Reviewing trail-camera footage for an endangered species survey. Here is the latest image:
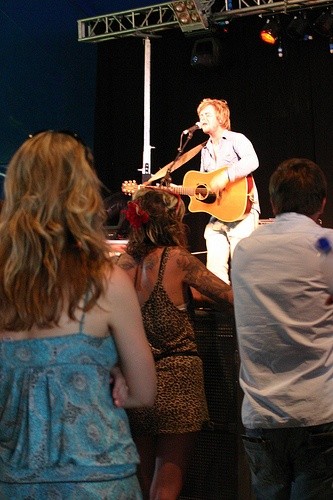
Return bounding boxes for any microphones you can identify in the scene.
[183,122,202,134]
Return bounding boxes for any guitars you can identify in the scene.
[121,163,255,222]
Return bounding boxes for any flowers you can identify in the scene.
[120,199,149,227]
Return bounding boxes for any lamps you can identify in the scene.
[169,0,210,33]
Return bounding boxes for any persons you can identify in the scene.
[156,98,261,286]
[231,160,333,500]
[0,132,156,500]
[116,188,234,500]
